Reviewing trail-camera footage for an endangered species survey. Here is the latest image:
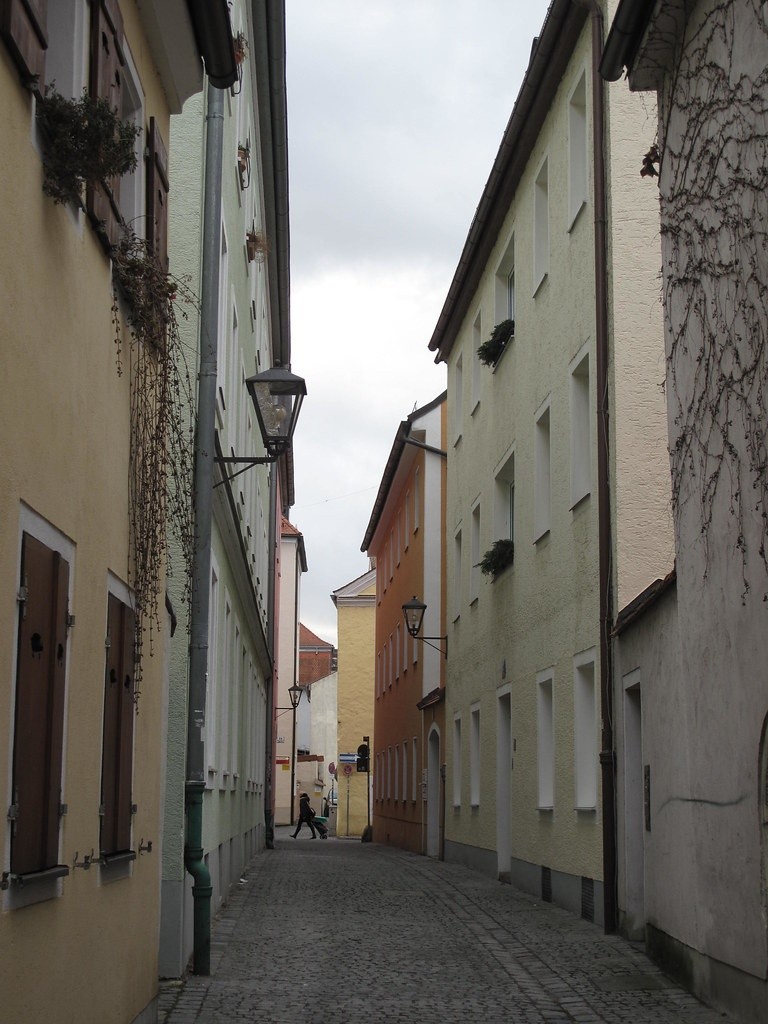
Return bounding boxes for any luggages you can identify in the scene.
[312,821,328,839]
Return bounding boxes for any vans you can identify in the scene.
[324,787,339,816]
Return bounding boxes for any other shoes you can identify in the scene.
[290,835,296,838]
[310,836,316,840]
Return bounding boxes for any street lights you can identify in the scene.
[287,681,302,825]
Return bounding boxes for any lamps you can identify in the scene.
[214,357,308,495]
[402,595,448,659]
[273,681,303,717]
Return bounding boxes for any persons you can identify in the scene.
[289,791,317,840]
